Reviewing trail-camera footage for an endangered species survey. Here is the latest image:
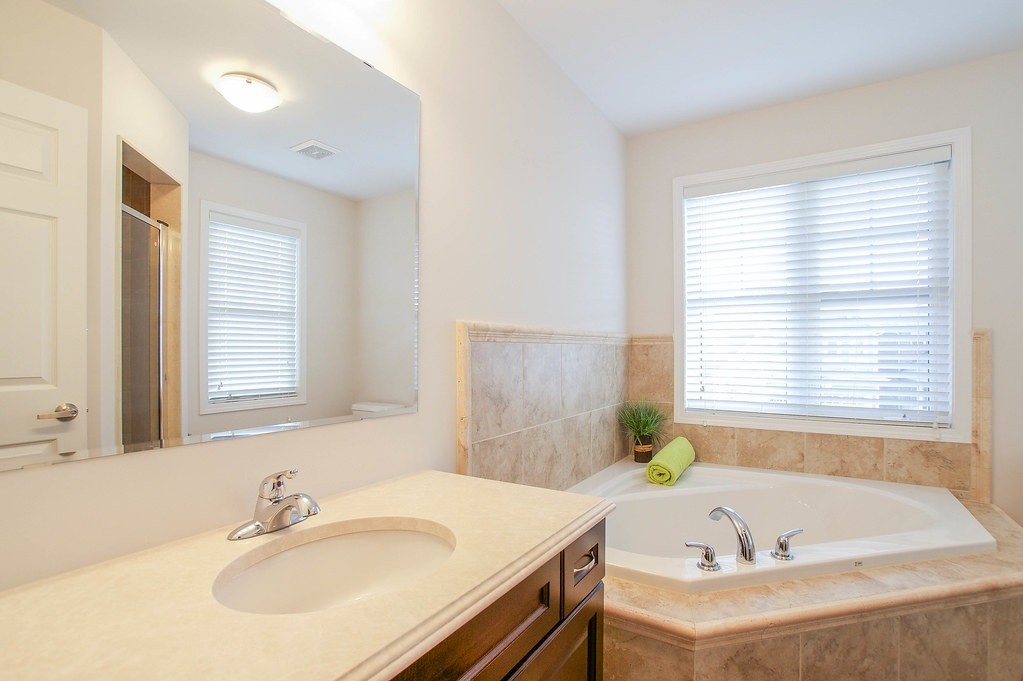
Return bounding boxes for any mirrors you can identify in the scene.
[0,0,424,470]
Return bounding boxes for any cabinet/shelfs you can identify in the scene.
[390,518,604,681]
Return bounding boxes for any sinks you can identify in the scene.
[211,516,456,614]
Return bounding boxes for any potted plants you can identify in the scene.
[617,396,669,464]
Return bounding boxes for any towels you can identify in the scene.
[646,436,695,485]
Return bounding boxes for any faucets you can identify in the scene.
[707,506,757,565]
[227,468,321,541]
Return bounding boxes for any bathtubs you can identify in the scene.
[561,455,997,594]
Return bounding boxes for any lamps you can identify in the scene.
[214,70,283,116]
